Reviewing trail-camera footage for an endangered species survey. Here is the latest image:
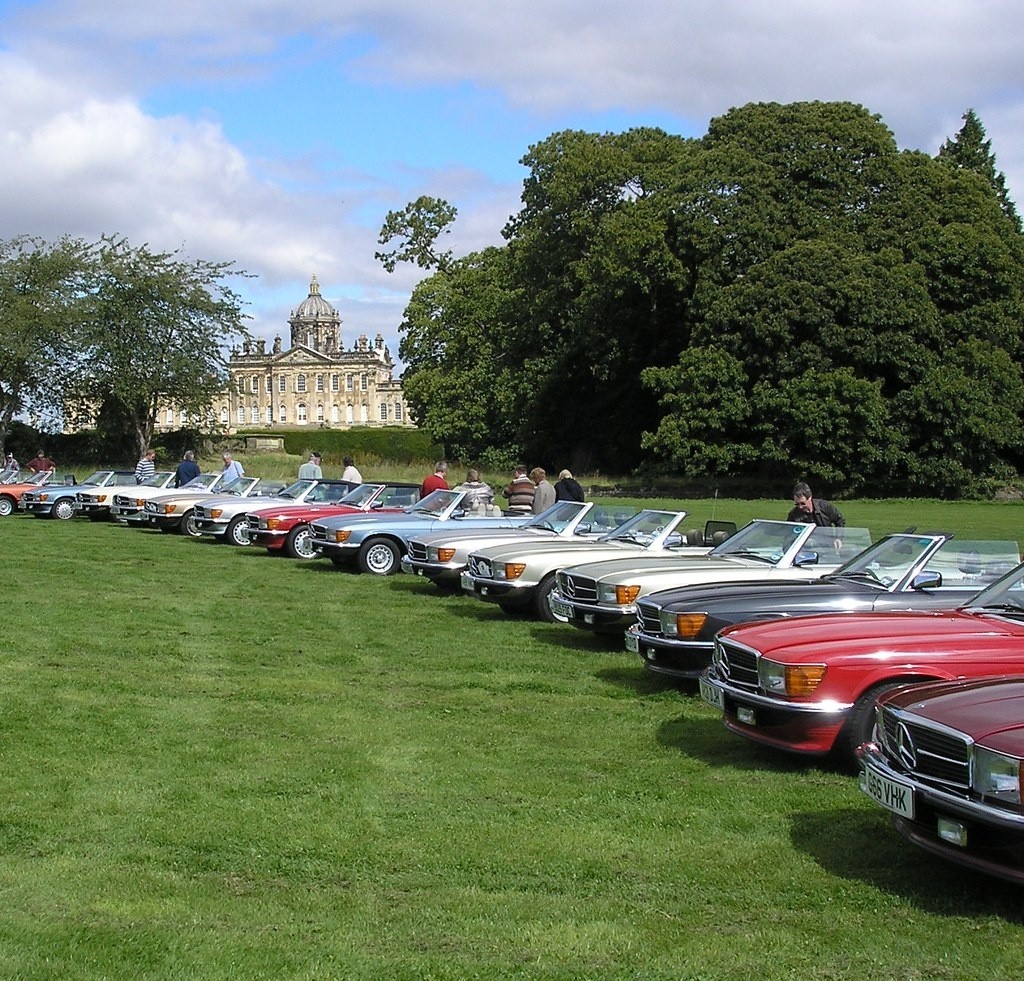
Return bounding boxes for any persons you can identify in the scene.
[26,450,55,472]
[2,452,20,471]
[420,461,449,508]
[342,457,362,484]
[783,482,846,556]
[134,449,155,483]
[458,469,495,508]
[175,451,200,487]
[502,464,584,521]
[222,452,245,483]
[298,452,326,497]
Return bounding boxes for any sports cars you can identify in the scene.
[459,507,783,623]
[547,520,912,641]
[624,526,1023,686]
[698,562,1024,774]
[0,459,605,620]
[855,675,1023,882]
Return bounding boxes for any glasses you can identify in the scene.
[794,502,806,505]
[152,456,155,458]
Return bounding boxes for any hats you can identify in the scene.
[310,451,320,458]
